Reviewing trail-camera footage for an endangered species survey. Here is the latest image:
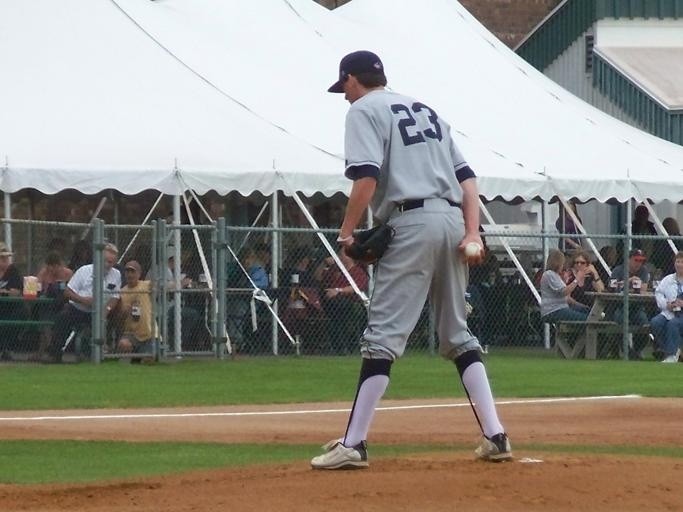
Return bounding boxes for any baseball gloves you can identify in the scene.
[345,224,394,262]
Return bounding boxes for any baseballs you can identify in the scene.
[465,242,480,257]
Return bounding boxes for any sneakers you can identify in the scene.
[475,433,512,461]
[28,352,62,361]
[119,358,131,362]
[311,438,370,470]
[631,347,645,359]
[661,348,681,363]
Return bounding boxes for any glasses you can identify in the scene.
[576,261,587,265]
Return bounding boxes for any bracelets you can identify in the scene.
[337,235,353,246]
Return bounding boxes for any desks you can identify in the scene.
[0,287,660,365]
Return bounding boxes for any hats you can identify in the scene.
[629,249,646,259]
[124,261,142,272]
[328,51,383,93]
[0,241,14,256]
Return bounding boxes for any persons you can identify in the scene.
[309,50,513,471]
[465,207,682,366]
[0,203,370,365]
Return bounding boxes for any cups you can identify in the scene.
[290,274,299,282]
[609,279,618,293]
[633,280,642,294]
